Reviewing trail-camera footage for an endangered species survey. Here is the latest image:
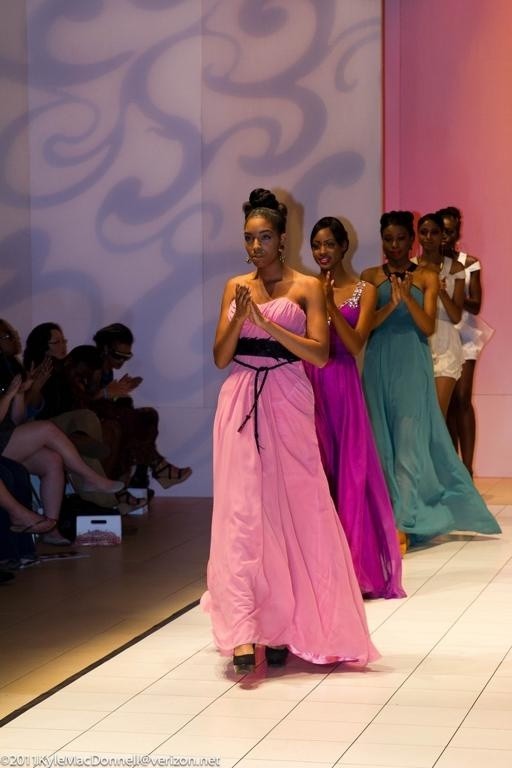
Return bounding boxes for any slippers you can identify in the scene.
[8,514,58,535]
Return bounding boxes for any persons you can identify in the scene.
[200,186,502,671]
[0,318,192,584]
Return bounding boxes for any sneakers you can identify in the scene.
[40,526,71,546]
[70,473,126,495]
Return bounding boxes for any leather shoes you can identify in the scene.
[232,643,256,675]
[264,644,289,665]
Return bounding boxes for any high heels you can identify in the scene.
[114,486,148,516]
[151,462,193,490]
